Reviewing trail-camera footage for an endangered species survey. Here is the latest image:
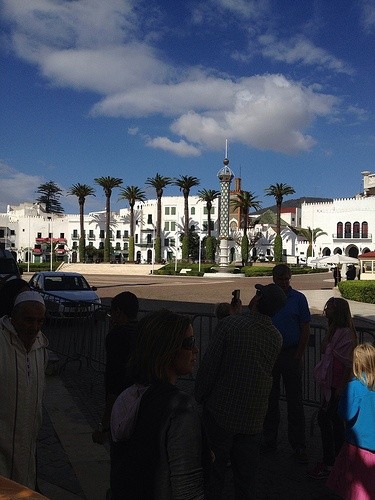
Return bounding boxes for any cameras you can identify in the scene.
[232,289,240,303]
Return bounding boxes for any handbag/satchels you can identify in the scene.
[109,385,150,443]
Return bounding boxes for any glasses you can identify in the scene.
[182,336,196,350]
[256,289,261,296]
[330,297,336,306]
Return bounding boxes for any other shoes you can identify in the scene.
[261,439,277,455]
[288,448,308,464]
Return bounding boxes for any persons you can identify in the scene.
[110,308,208,500]
[197,283,287,500]
[249,264,311,465]
[105,291,140,439]
[326,343,375,500]
[0,278,49,491]
[305,297,359,480]
[333,265,361,287]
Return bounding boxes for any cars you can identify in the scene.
[27,271,102,320]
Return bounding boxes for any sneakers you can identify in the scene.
[305,463,333,480]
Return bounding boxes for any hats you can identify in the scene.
[14,291,46,306]
[255,284,287,308]
